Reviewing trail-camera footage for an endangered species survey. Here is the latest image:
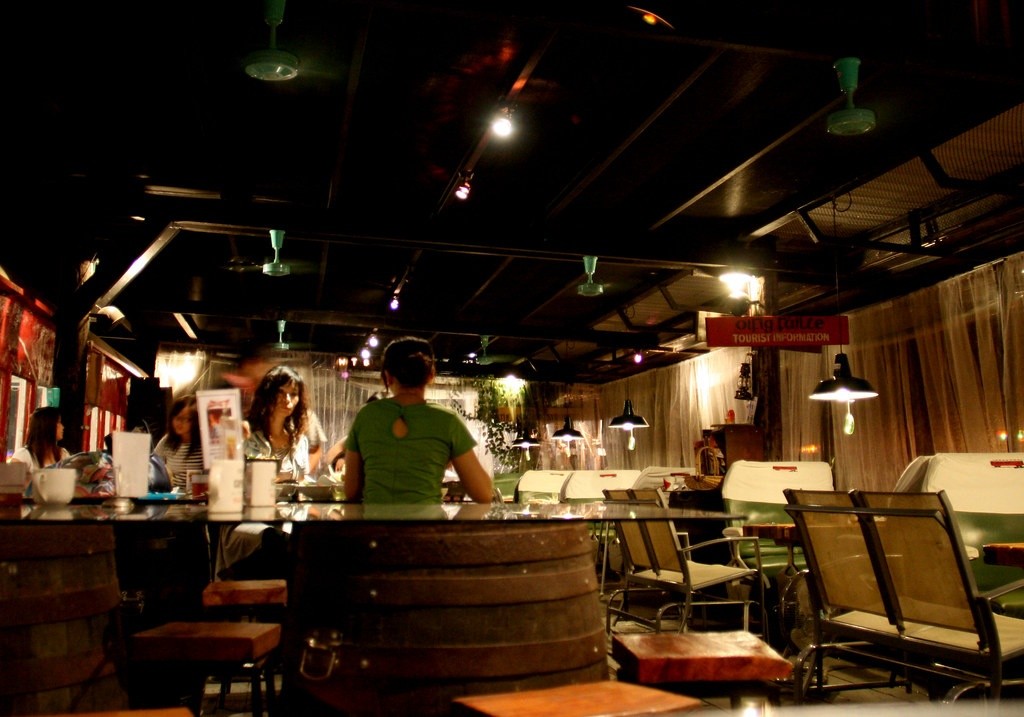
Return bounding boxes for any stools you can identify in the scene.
[611,631,793,711]
[131,578,290,717]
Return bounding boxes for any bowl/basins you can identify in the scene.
[293,482,332,500]
[276,483,298,502]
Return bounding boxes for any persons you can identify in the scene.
[216,366,327,625]
[344,338,492,504]
[342,504,491,520]
[154,396,210,619]
[333,451,347,471]
[11,406,70,496]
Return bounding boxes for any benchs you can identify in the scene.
[885,451,1024,620]
[721,460,844,616]
[513,466,695,544]
[783,488,1024,706]
[598,489,767,635]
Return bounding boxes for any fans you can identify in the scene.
[223,229,321,277]
[552,256,637,297]
[211,0,340,82]
[254,320,310,353]
[826,59,878,139]
[460,336,526,365]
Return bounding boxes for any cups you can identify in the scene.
[192,474,209,499]
[186,470,202,494]
[245,461,277,521]
[31,468,77,503]
[209,458,244,521]
[331,483,348,501]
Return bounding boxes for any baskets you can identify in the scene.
[686,447,723,490]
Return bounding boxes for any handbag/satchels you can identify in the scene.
[135,419,174,492]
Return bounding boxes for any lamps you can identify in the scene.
[809,190,879,435]
[93,303,126,332]
[734,352,755,402]
[551,406,585,457]
[512,417,541,461]
[391,297,399,309]
[608,371,649,451]
[456,179,471,200]
